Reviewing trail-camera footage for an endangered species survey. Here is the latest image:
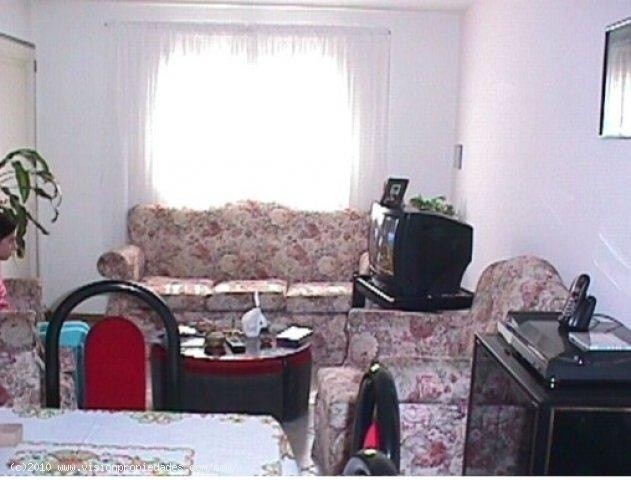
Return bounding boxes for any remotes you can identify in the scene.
[227,336,247,355]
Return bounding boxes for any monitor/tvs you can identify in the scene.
[367,201,474,301]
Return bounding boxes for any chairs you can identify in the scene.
[44,279,181,411]
[0,277,77,410]
[340,364,399,476]
[311,254,569,475]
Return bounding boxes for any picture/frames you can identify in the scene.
[380,179,409,208]
[598,16,631,139]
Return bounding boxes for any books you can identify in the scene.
[569,327,631,355]
[277,325,314,349]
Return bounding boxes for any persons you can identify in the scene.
[0,211,19,407]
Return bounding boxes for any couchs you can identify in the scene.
[98,205,369,366]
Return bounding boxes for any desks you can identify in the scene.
[0,407,297,476]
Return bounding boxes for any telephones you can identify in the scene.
[557,274,597,332]
